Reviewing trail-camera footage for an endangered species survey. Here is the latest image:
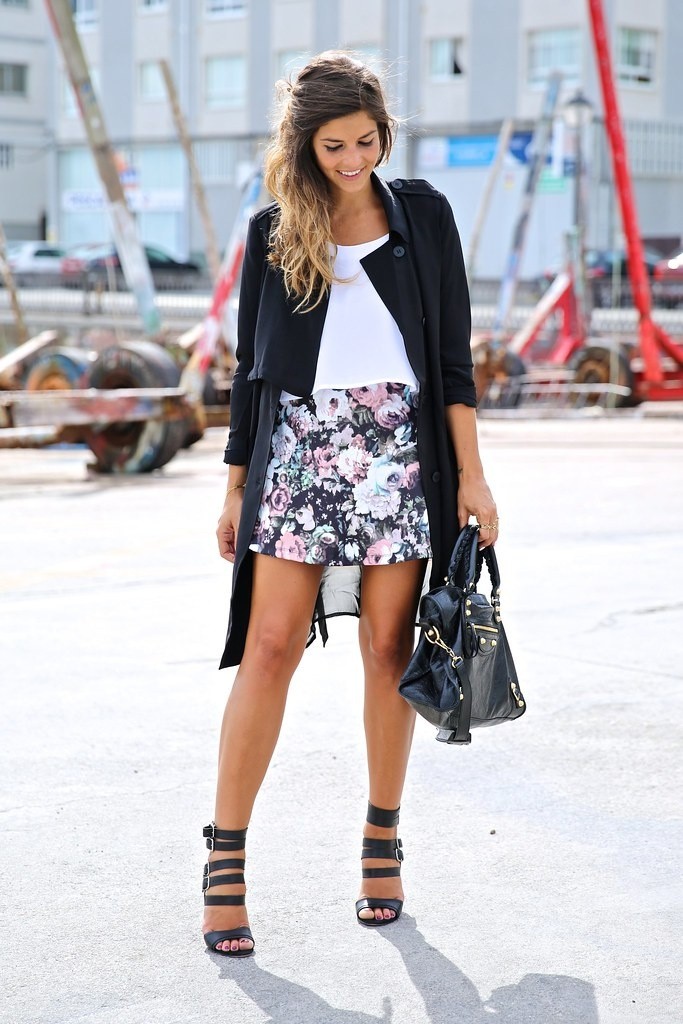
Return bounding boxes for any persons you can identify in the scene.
[200,52,500,959]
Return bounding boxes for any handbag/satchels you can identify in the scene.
[396,524,526,745]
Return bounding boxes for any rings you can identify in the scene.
[479,522,497,531]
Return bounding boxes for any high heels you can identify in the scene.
[203,821,255,958]
[356,801,404,927]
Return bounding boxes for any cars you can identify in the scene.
[541,250,662,308]
[0,240,71,287]
[62,242,199,293]
[653,248,683,283]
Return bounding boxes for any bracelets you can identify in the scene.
[225,483,246,497]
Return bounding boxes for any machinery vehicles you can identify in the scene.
[0,0,683,473]
